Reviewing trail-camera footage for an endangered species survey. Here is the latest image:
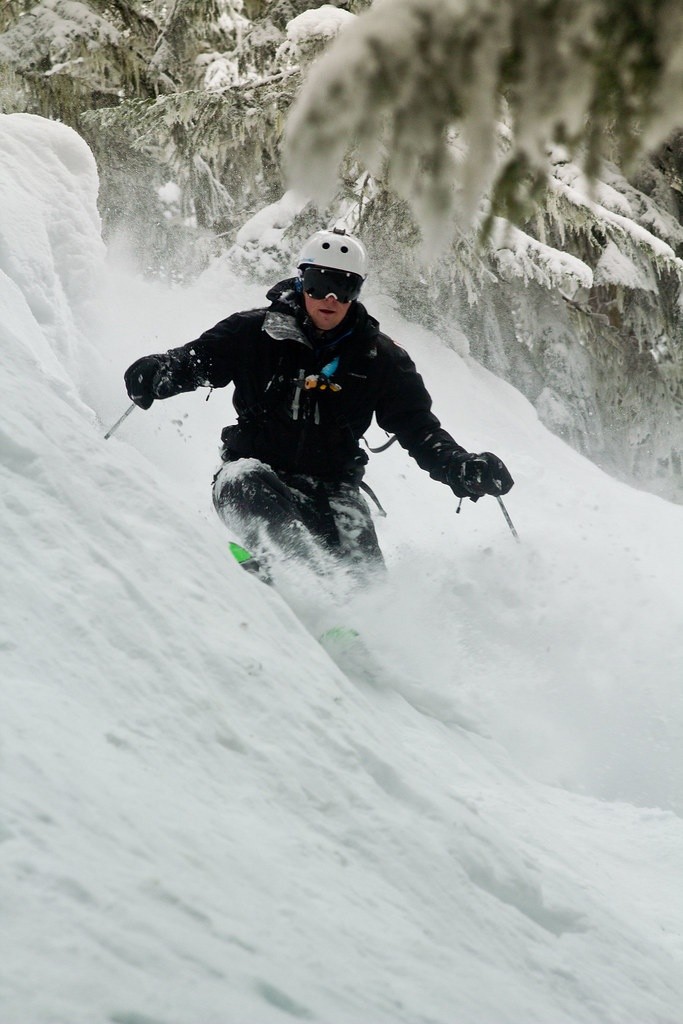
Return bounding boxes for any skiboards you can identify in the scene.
[229,540,279,592]
[315,622,386,682]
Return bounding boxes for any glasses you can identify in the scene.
[299,268,364,304]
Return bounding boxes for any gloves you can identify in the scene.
[123,357,171,410]
[448,447,515,515]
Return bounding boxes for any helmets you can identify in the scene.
[296,226,368,280]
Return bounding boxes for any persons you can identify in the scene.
[125,228,514,591]
